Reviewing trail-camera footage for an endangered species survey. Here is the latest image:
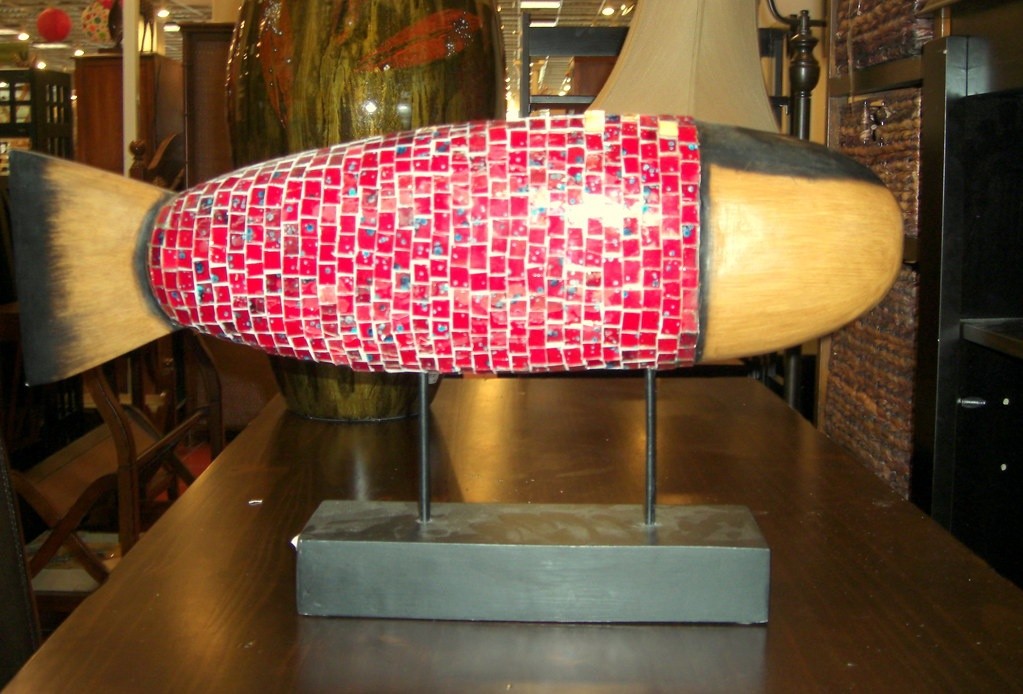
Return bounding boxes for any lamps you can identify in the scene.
[602,1,614,16]
[584,0,780,135]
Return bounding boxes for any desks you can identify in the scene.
[0,376,1023,694]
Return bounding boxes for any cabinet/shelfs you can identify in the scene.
[0,52,158,182]
